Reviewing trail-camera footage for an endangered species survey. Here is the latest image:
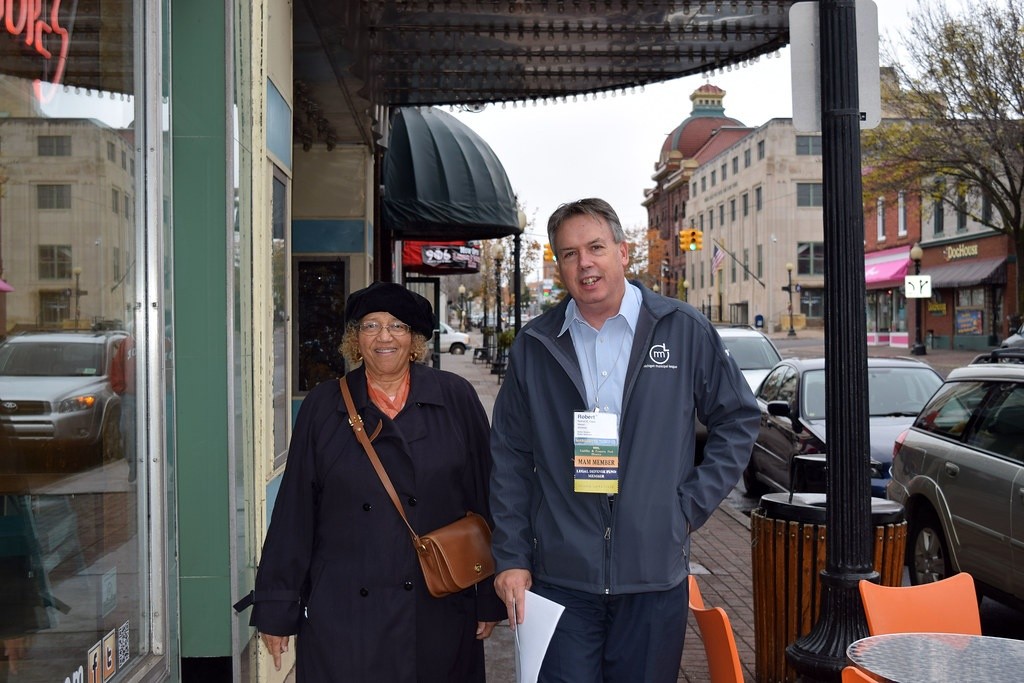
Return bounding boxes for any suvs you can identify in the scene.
[711,322,784,395]
[0,329,131,462]
[886,347,1024,611]
[741,357,974,498]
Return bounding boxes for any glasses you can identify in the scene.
[354,319,412,337]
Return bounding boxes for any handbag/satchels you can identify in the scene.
[417,512,496,598]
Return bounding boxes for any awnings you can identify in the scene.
[401,240,483,275]
[918,256,1008,288]
[386,104,519,240]
[864,244,913,288]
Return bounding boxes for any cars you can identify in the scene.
[427,322,472,356]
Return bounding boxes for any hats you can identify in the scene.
[345,280,434,342]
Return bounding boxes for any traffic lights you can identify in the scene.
[688,230,697,251]
[679,230,688,251]
[696,231,704,250]
[544,242,554,262]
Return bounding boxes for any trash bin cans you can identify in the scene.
[755,314,764,331]
[781,312,807,331]
[749,492,908,683]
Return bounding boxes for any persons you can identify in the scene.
[0,402,54,682]
[111,319,170,483]
[250,281,508,683]
[487,197,761,683]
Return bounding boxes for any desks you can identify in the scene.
[846,633,1024,683]
[30,456,136,640]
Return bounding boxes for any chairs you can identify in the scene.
[987,405,1024,454]
[858,572,983,635]
[689,574,744,683]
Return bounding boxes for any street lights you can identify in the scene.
[910,242,926,354]
[785,263,796,336]
[458,284,466,333]
[74,267,82,329]
[490,243,508,374]
[513,202,527,337]
